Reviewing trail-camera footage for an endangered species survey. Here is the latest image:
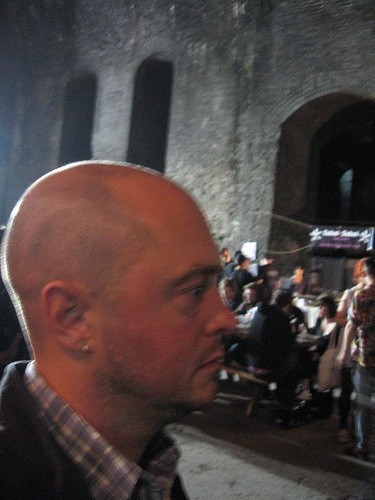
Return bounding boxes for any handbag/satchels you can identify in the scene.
[317,318,346,392]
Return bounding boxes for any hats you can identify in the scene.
[269,289,293,310]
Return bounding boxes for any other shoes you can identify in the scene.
[338,427,350,443]
[311,411,331,421]
[295,384,313,401]
[271,398,306,418]
[344,445,367,459]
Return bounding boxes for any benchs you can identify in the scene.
[218,363,268,416]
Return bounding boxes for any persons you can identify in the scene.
[0,159,237,500]
[217,240,375,463]
